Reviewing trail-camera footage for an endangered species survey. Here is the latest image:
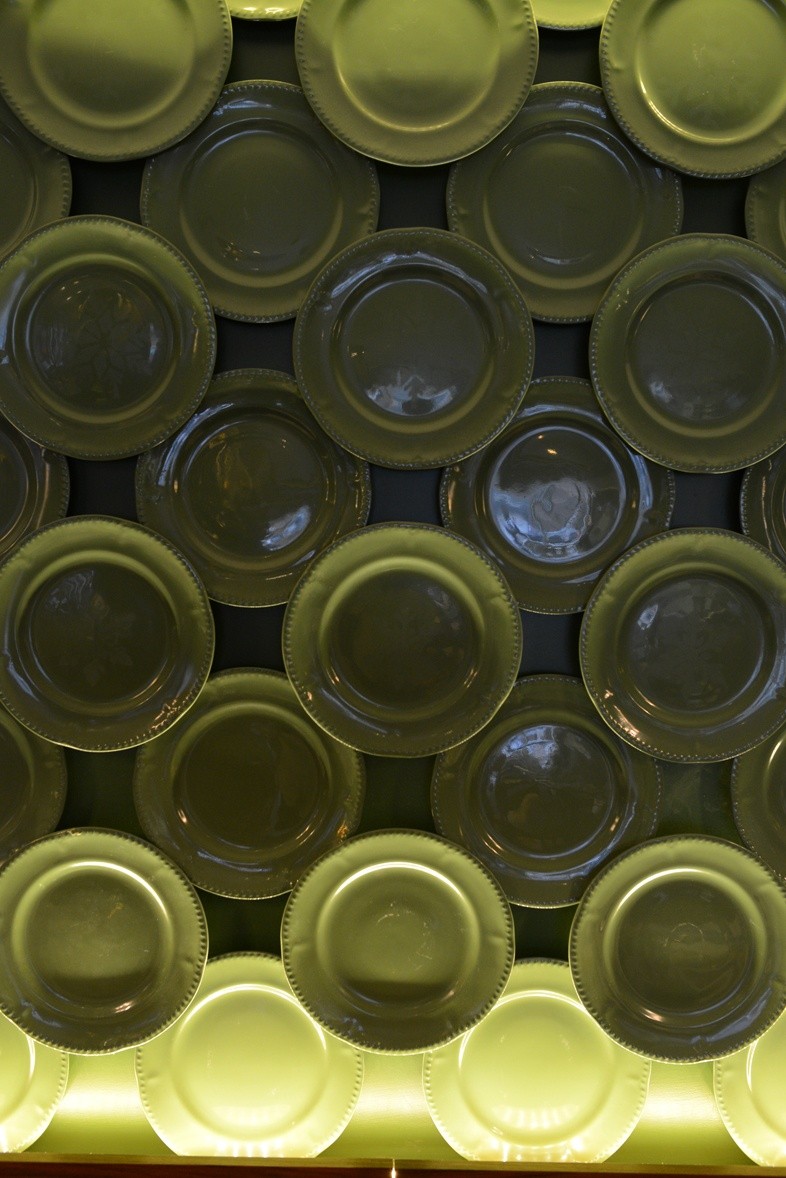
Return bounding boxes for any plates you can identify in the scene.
[139,78,381,322]
[291,227,537,469]
[422,958,651,1163]
[0,0,785,177]
[445,80,683,325]
[429,675,663,909]
[279,827,516,1057]
[440,376,676,615]
[135,951,364,1159]
[132,667,365,901]
[280,521,523,758]
[0,93,216,1155]
[713,723,786,1169]
[568,833,786,1065]
[589,161,786,565]
[578,526,786,764]
[133,368,371,608]
